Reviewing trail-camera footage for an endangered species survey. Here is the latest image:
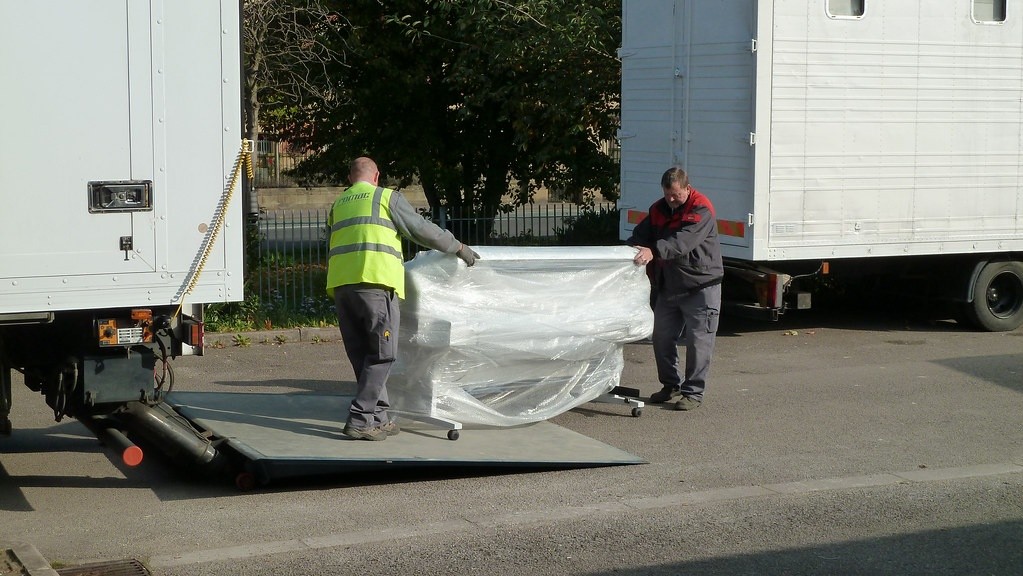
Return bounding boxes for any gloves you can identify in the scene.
[456,243,481,268]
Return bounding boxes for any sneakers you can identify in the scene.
[343,421,387,441]
[373,420,399,436]
[649,385,683,403]
[674,396,700,411]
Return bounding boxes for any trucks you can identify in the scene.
[615,0,1023,332]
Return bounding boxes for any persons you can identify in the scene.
[325,156,479,442]
[623,167,723,410]
